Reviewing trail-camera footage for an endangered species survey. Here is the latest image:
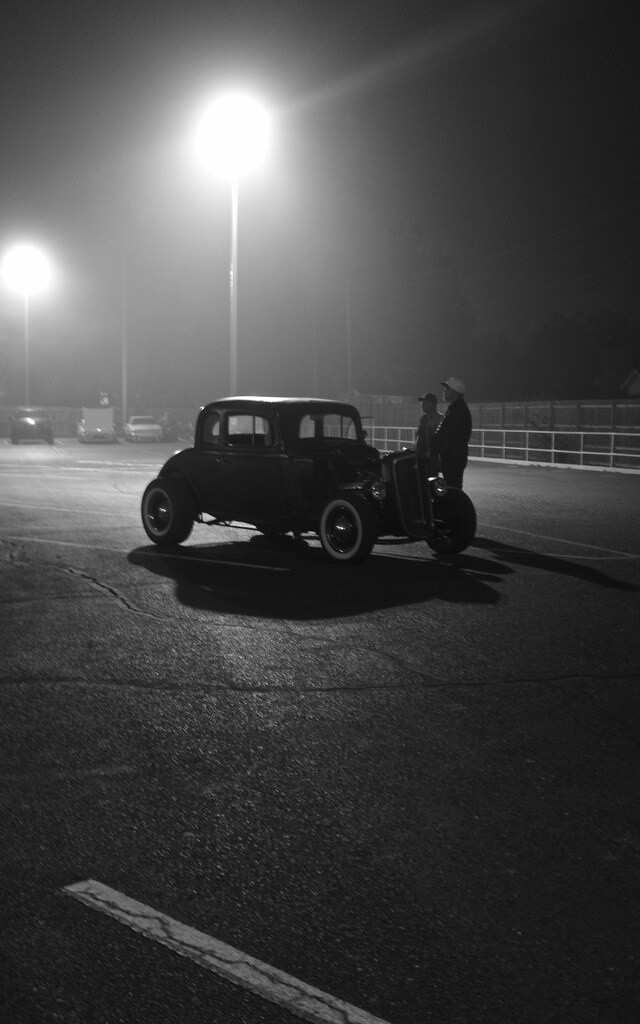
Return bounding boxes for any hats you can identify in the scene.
[440,377,465,394]
[418,393,437,401]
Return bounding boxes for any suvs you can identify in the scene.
[9,408,55,445]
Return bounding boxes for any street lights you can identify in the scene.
[1,243,51,406]
[184,85,272,395]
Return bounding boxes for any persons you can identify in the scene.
[414,392,445,477]
[429,376,472,490]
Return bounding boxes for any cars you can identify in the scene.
[124,415,162,443]
[77,406,117,444]
[141,394,480,564]
[159,413,193,439]
